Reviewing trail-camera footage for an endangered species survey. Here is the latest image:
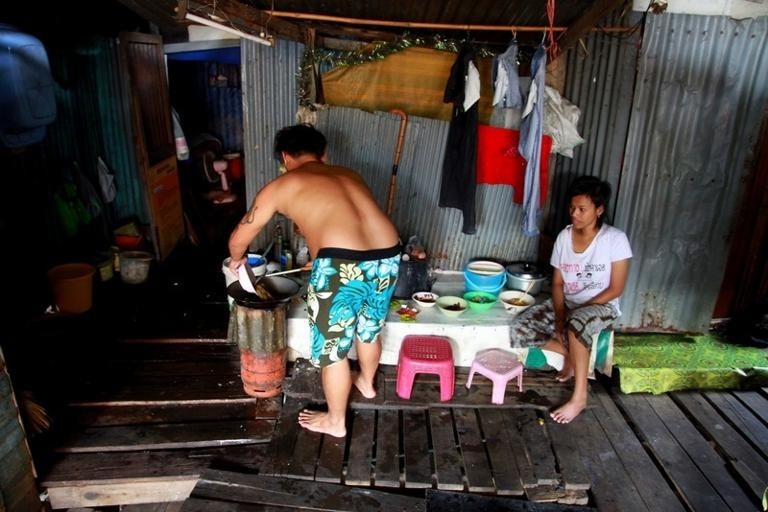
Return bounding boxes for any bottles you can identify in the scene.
[285,251,292,270]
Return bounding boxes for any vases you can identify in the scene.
[47,250,154,315]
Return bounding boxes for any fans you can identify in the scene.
[198,145,238,204]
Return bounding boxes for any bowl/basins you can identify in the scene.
[239,263,258,294]
[412,290,536,318]
[114,233,143,248]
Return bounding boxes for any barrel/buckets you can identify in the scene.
[463,261,507,299]
[48,262,95,314]
[221,254,267,312]
[392,261,428,301]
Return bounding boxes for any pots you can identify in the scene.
[227,275,299,309]
[506,264,549,296]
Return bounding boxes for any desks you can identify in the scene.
[285,299,552,366]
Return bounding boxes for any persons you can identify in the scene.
[228,122,402,437]
[509,175,634,424]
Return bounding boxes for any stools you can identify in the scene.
[395,335,525,404]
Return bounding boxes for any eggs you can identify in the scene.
[418,251,426,259]
